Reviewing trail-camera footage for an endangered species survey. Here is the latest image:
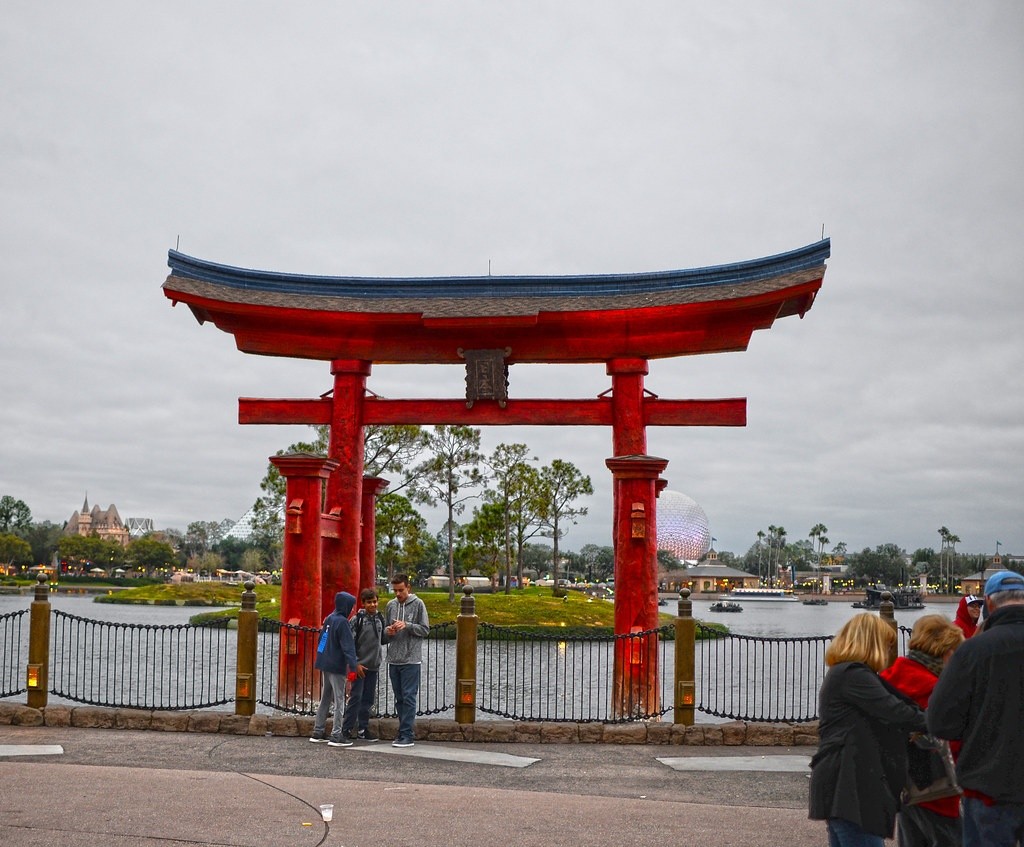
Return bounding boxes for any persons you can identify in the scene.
[309,591,367,746]
[385,573,430,747]
[235,569,245,581]
[878,613,967,847]
[341,588,388,743]
[808,612,928,847]
[950,593,985,640]
[928,570,1024,847]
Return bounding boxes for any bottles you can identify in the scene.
[265,729,272,747]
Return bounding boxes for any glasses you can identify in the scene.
[970,604,982,608]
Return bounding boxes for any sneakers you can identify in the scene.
[327,736,354,746]
[357,729,379,742]
[309,735,330,742]
[391,736,414,747]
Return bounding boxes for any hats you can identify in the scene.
[983,572,1024,597]
[965,595,984,605]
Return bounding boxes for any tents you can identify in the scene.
[465,577,490,587]
[427,576,450,589]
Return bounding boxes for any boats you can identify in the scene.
[709,602,744,612]
[803,598,827,606]
[850,584,927,610]
[658,597,668,606]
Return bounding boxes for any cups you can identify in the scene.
[320,804,334,821]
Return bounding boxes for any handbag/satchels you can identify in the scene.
[901,732,962,805]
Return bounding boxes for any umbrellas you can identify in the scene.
[30,564,54,573]
[90,567,105,575]
[115,568,125,573]
